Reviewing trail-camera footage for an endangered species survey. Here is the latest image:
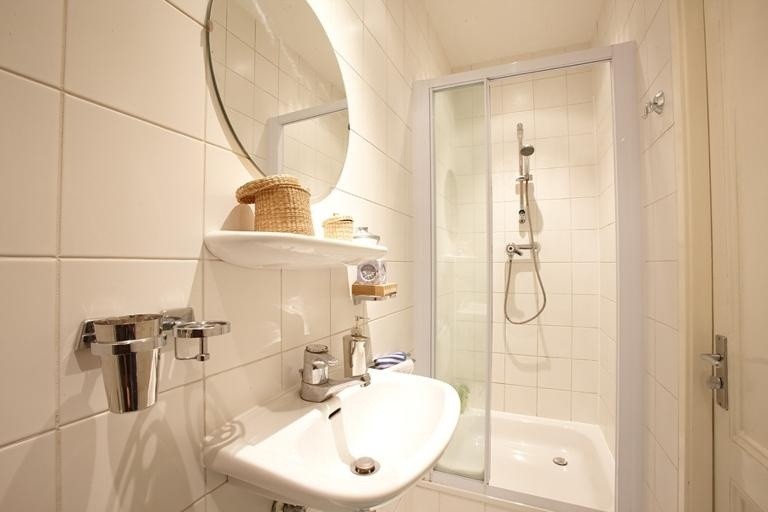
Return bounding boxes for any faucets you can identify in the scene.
[300,344,375,401]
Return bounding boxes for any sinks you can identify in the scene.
[203,368,462,510]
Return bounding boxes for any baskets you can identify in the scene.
[322,215,355,240]
[235,175,315,234]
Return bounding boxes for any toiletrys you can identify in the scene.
[341,316,369,378]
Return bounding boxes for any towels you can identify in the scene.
[372,351,414,376]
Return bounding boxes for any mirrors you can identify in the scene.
[206,0,350,206]
